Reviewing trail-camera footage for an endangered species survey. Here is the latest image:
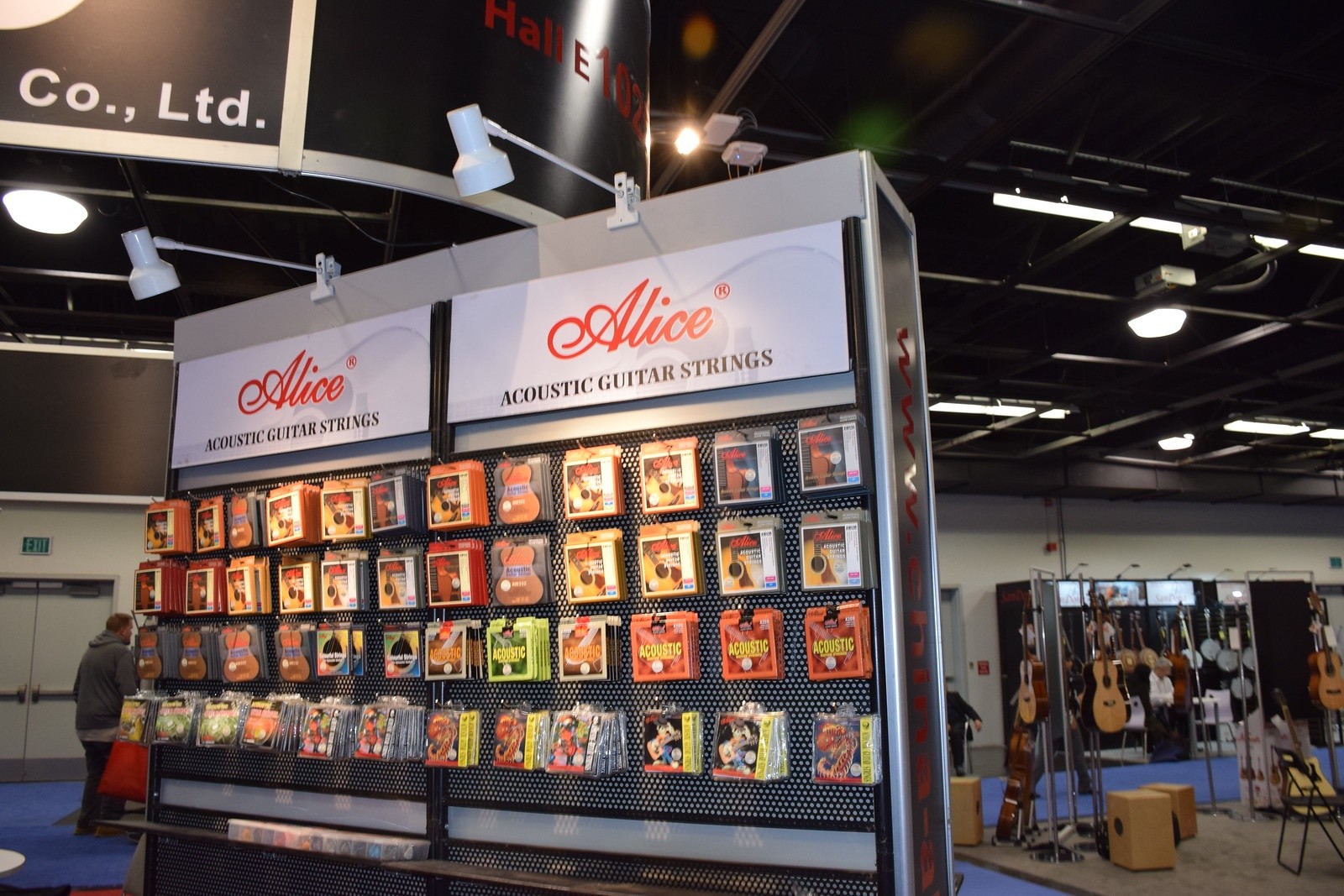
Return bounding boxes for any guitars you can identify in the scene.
[1270,685,1339,816]
[1017,587,1052,725]
[1100,594,1133,727]
[1118,600,1261,712]
[1081,588,1127,736]
[994,719,1039,844]
[1306,588,1344,711]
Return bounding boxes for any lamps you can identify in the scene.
[447,102,642,229]
[121,225,341,301]
[2,189,88,233]
[928,192,1344,451]
[673,118,708,155]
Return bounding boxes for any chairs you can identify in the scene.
[1271,746,1344,877]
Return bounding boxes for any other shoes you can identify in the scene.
[74,827,92,835]
[956,766,965,776]
[94,824,126,837]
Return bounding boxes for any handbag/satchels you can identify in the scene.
[96,739,149,804]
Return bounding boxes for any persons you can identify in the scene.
[944,677,982,777]
[1149,657,1175,731]
[1018,652,1099,800]
[1104,584,1128,607]
[72,613,140,836]
